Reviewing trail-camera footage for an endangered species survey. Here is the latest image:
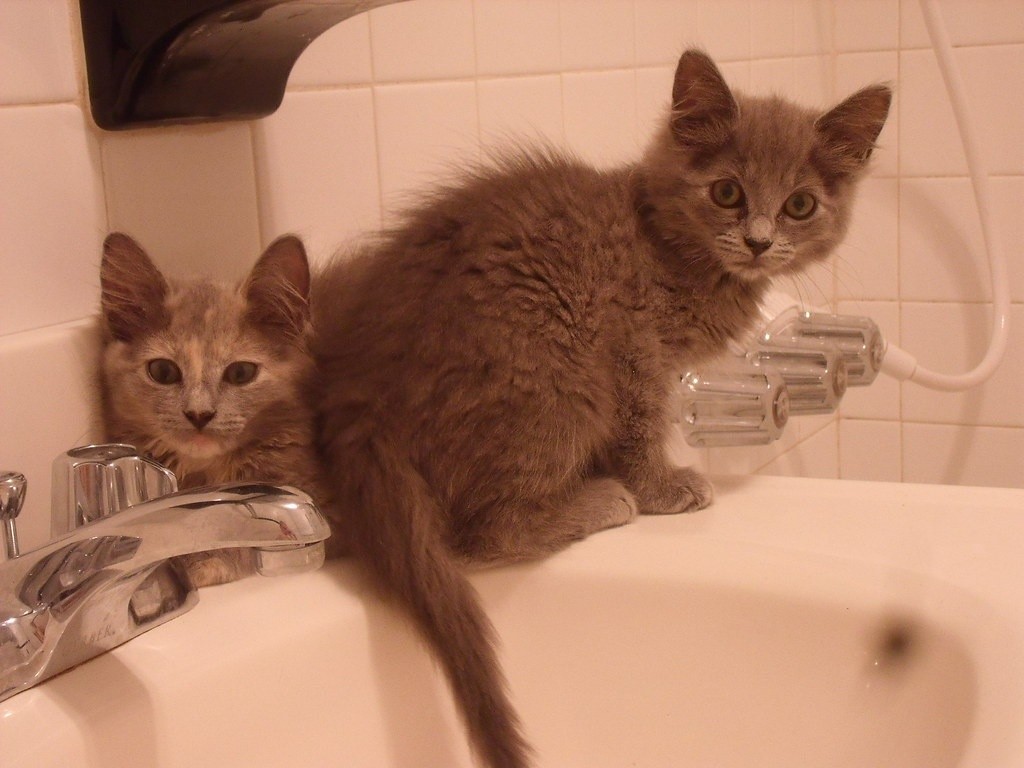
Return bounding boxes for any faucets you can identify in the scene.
[1,476,330,694]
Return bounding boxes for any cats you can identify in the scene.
[310,46,897,768]
[88,228,310,583]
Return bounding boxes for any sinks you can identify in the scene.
[0,469,1023,768]
